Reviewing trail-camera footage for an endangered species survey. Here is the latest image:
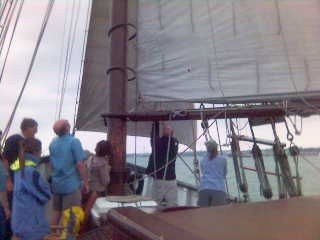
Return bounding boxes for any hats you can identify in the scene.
[205,141,218,149]
[53,120,70,136]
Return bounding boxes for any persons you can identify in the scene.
[142,109,179,211]
[0,131,22,222]
[199,139,227,207]
[11,138,56,240]
[84,140,111,199]
[49,119,88,232]
[21,118,40,151]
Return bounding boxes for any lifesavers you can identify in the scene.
[126,171,145,195]
